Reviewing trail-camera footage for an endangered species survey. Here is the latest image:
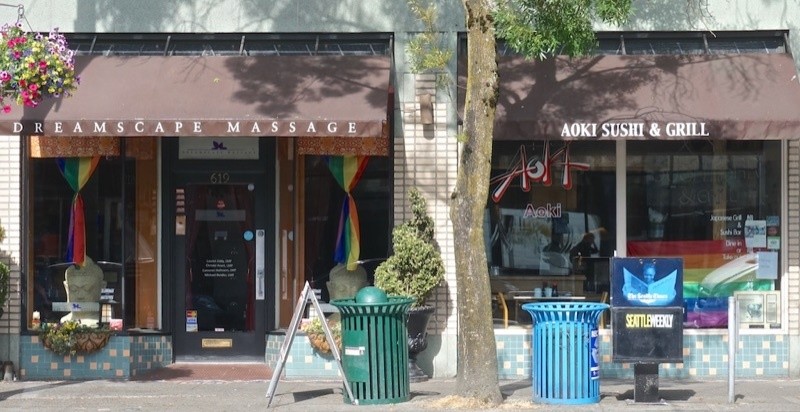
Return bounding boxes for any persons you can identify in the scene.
[569,233,599,289]
[622,263,677,304]
[59,255,107,327]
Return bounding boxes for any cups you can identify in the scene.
[552,286,558,297]
[534,286,543,297]
[544,286,553,297]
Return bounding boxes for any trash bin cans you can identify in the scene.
[329,287,416,405]
[522,301,610,406]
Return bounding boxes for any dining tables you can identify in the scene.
[510,287,585,324]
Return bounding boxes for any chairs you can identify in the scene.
[596,290,609,327]
[492,291,515,328]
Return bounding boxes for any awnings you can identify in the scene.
[455,52,799,140]
[0,56,391,137]
